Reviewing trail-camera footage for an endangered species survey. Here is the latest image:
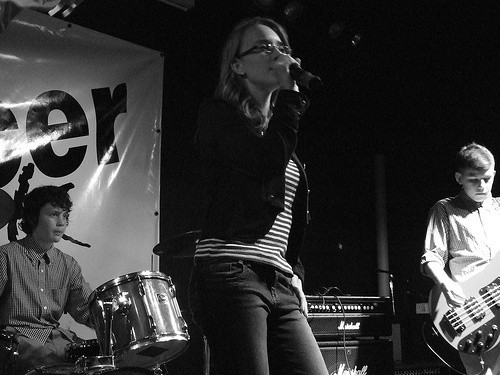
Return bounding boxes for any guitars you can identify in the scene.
[428,273,500,355]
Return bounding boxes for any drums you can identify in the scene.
[87,365,164,375]
[86,271,191,368]
[0,329,19,375]
[24,364,89,375]
[63,339,104,373]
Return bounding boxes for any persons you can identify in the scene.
[187,16,329,375]
[0,185,94,375]
[420,142,500,375]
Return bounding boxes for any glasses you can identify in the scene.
[238,43,292,60]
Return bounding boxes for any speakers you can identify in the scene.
[316,339,396,375]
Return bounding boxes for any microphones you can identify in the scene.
[289,64,323,92]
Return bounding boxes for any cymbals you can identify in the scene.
[153,229,201,256]
